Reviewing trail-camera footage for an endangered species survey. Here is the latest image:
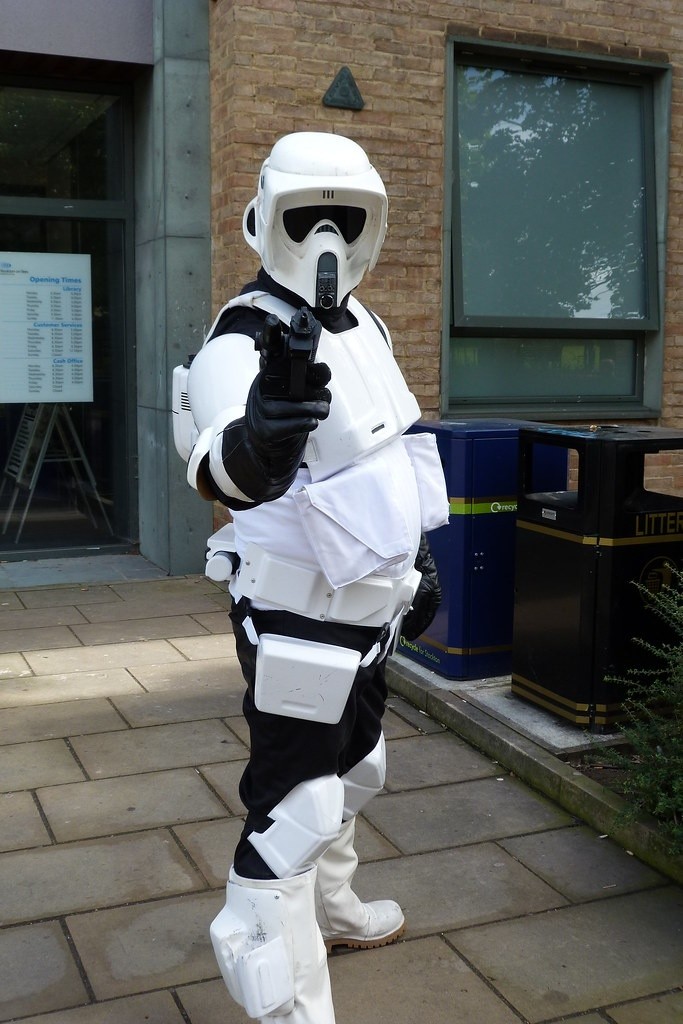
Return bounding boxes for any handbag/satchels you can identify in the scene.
[240,608,406,725]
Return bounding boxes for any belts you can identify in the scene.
[233,542,422,628]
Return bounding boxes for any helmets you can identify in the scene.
[242,131,390,312]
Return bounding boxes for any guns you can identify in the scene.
[254,306,323,401]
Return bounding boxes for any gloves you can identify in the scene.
[220,340,332,504]
[397,531,442,641]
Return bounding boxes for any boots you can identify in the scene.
[210,860,336,1024]
[313,813,406,953]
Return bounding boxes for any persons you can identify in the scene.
[187,132,450,1024]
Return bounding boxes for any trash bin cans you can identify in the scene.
[512,423,683,731]
[401,419,569,683]
